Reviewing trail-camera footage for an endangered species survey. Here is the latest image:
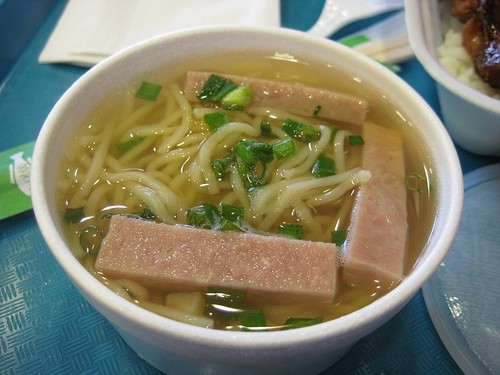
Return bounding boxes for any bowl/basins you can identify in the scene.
[29,24,464,375]
[405,0,500,157]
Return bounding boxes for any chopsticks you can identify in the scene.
[348,35,413,65]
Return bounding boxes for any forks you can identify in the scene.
[304,0,403,40]
